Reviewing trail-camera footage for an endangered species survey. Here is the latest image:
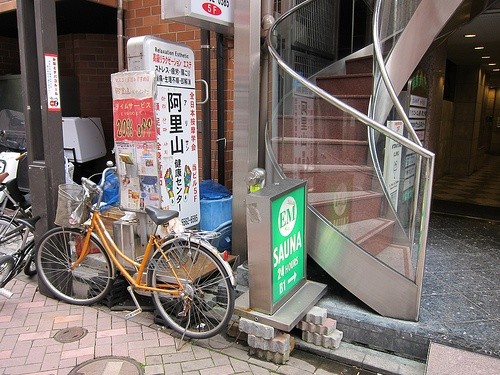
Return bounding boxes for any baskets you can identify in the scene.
[53,184,91,227]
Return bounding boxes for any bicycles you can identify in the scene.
[0,213,40,288]
[34,161,238,351]
[0,170,32,248]
